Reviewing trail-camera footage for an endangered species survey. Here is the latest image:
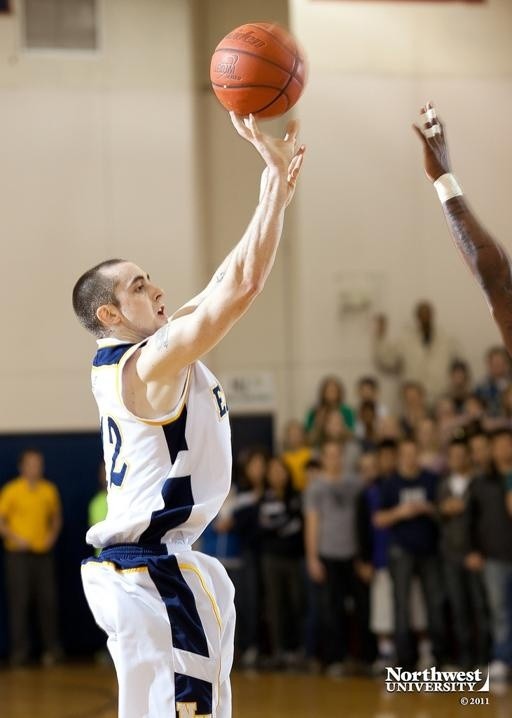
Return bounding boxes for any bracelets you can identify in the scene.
[431,171,464,202]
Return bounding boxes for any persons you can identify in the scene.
[0,453,60,671]
[72,112,307,718]
[410,100,512,351]
[82,457,114,665]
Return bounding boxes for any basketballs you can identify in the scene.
[209,22,308,121]
